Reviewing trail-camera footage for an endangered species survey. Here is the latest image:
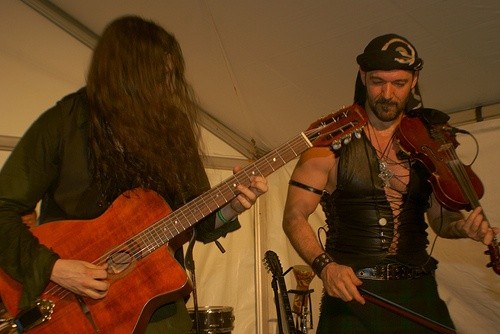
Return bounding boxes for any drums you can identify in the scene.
[187,305,235,334]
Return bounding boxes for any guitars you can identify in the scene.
[261,248,298,334]
[0,102,371,334]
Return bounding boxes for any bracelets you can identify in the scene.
[217,210,229,224]
[311,252,337,279]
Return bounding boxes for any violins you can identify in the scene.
[396,106,500,276]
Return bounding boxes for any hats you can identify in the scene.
[356,33,424,73]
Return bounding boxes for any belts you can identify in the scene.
[357,263,433,282]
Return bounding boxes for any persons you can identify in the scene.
[282,34,500,334]
[0,14,269,334]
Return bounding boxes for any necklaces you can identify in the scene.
[368,120,397,187]
